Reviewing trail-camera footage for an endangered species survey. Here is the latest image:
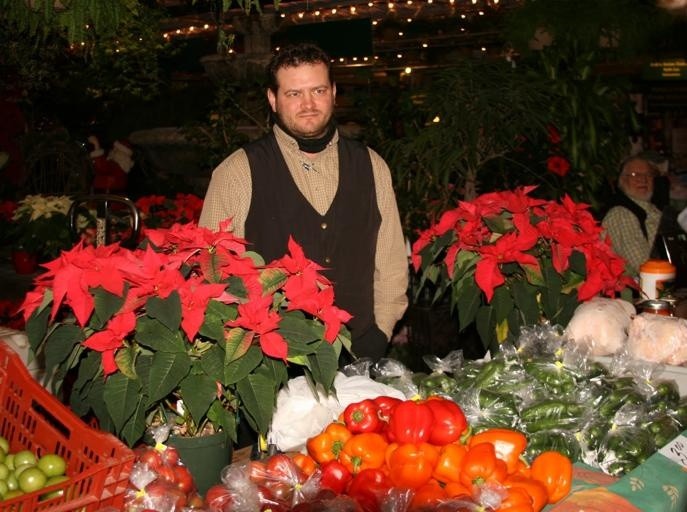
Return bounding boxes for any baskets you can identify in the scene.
[0,340,134,511]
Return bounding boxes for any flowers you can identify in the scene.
[10,220,357,452]
[410,185,642,360]
[1,193,206,259]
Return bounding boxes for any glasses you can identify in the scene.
[623,172,653,179]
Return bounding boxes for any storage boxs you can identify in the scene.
[561,351,687,399]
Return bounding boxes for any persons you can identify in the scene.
[601,155,670,271]
[71,113,133,196]
[643,126,687,247]
[197,41,409,450]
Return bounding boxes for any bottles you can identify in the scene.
[638,259,676,319]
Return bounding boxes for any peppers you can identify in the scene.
[343,327,686,475]
[305,396,575,512]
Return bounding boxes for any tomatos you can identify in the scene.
[122,445,321,512]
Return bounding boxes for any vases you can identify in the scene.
[408,298,458,375]
[9,250,38,275]
[143,428,230,501]
